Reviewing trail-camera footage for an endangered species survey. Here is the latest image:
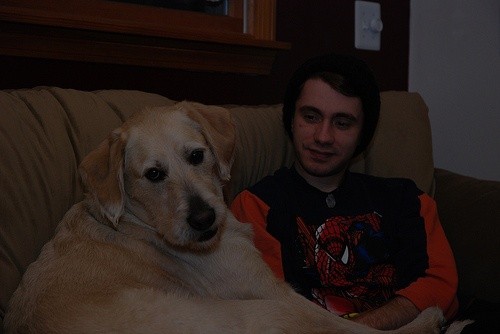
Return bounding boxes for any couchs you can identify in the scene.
[0,84,500,334]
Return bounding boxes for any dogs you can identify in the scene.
[3,100,475,334]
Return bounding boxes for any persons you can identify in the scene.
[235,41,458,334]
